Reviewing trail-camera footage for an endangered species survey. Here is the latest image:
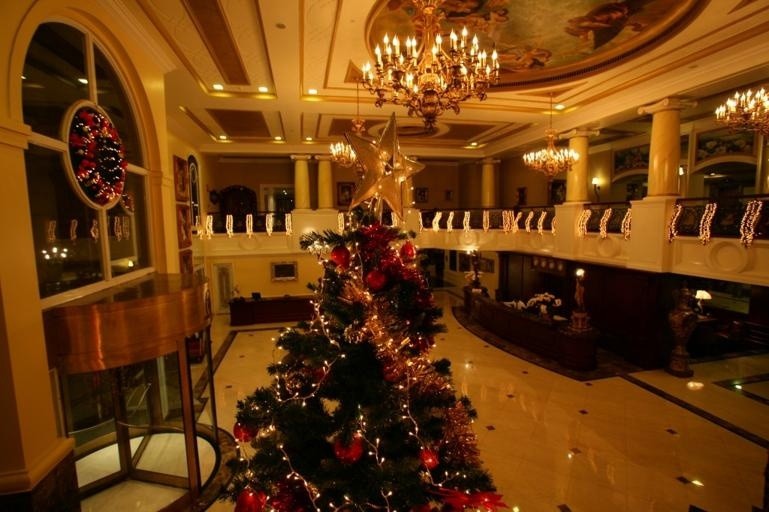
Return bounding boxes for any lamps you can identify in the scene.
[695,289,712,315]
[592,177,601,203]
[327,60,366,166]
[713,85,769,134]
[468,249,480,282]
[361,0,501,135]
[524,89,580,183]
[576,268,590,286]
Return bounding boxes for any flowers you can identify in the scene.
[527,291,562,312]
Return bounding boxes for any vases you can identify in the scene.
[540,312,552,321]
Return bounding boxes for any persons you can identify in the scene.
[441,0,510,34]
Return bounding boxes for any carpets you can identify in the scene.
[451,306,662,382]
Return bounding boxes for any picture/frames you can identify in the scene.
[174,154,194,290]
[337,181,356,206]
[548,179,566,205]
[416,187,429,202]
[271,260,299,282]
